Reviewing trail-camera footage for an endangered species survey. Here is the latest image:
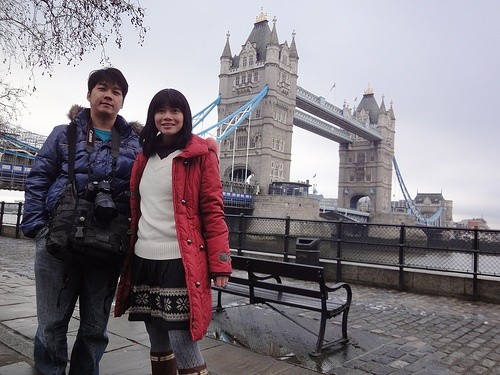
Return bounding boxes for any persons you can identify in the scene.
[20,67,144,375]
[114,90,232,375]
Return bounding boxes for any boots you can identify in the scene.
[148,350,178,375]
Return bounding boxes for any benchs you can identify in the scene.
[210,255,353,358]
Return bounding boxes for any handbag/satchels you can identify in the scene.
[45,191,131,268]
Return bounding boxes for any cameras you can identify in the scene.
[83,179,119,227]
